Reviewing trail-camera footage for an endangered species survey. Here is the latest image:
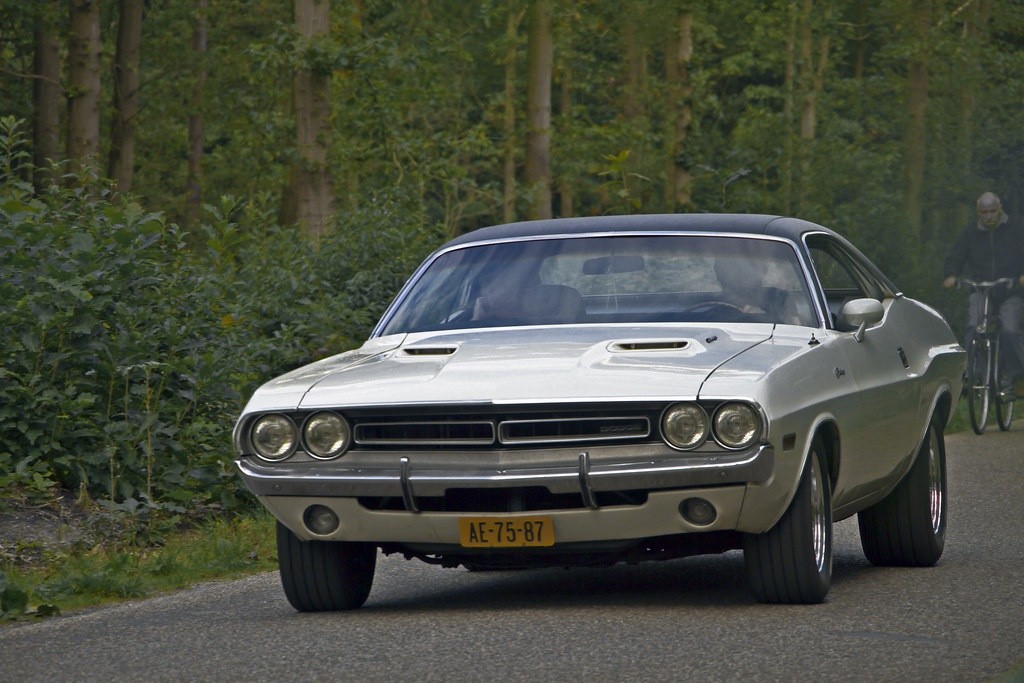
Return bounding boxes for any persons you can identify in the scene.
[449,262,548,323]
[711,255,799,326]
[944,192,1024,391]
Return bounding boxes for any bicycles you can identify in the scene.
[945,274,1016,435]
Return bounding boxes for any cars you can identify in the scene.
[229,212,969,611]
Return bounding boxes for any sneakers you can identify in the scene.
[998,380,1016,403]
